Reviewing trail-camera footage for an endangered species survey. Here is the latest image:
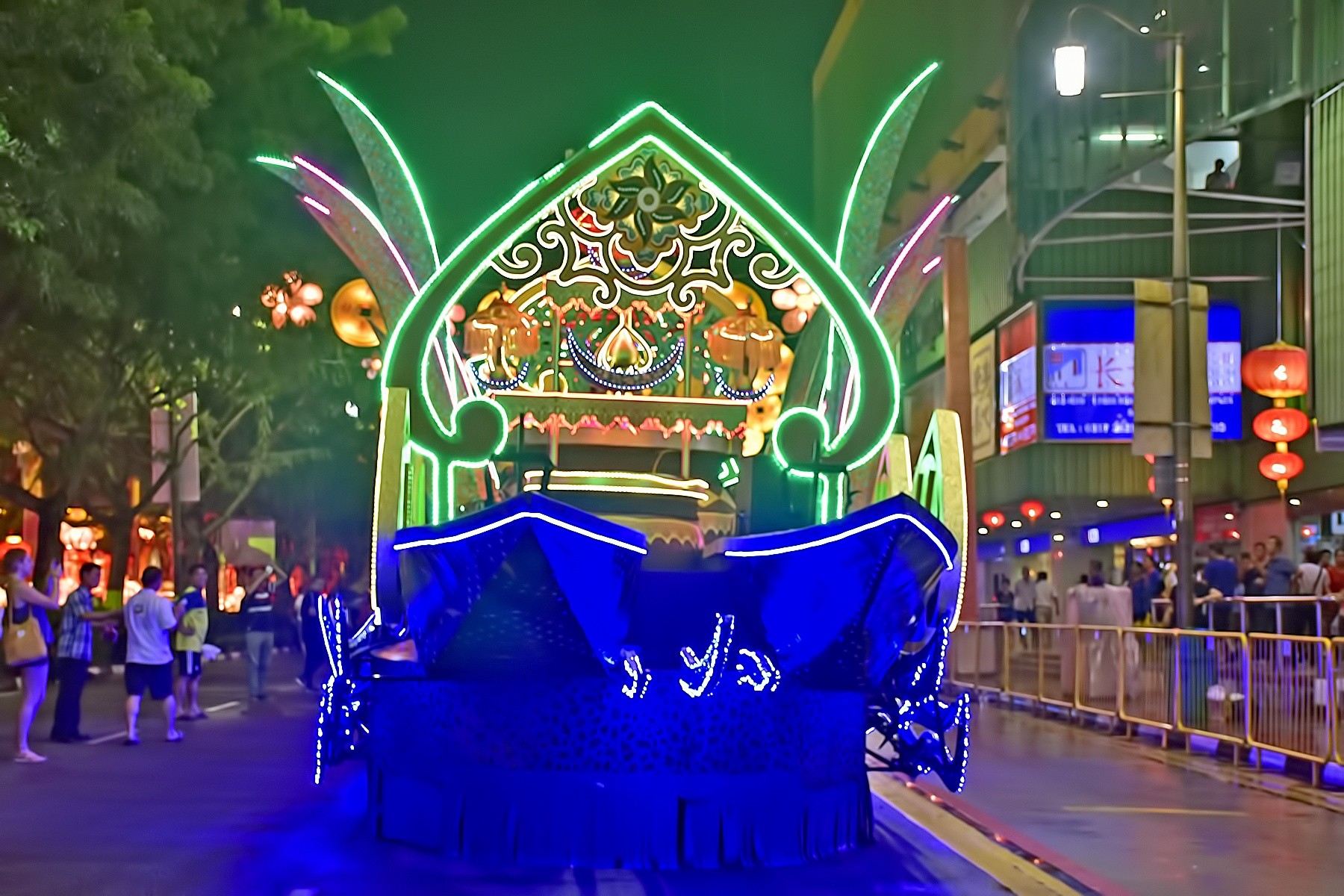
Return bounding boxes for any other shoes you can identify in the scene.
[15,747,45,762]
[306,687,320,693]
[52,734,88,744]
[187,713,209,724]
[296,676,306,687]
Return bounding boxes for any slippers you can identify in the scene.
[165,731,185,742]
[123,734,141,746]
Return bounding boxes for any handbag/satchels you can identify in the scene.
[3,581,48,667]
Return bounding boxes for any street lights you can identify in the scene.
[1050,0,1193,746]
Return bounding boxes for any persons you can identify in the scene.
[294,577,325,694]
[122,567,183,745]
[244,563,287,699]
[1241,536,1344,669]
[997,567,1059,649]
[1206,159,1230,190]
[51,563,119,744]
[173,565,209,720]
[0,548,63,762]
[1079,556,1178,647]
[1164,542,1245,664]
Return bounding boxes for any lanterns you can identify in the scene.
[1252,408,1309,453]
[1259,452,1304,494]
[0,536,33,563]
[982,511,1005,529]
[1145,454,1173,518]
[1241,341,1309,408]
[1021,500,1045,525]
